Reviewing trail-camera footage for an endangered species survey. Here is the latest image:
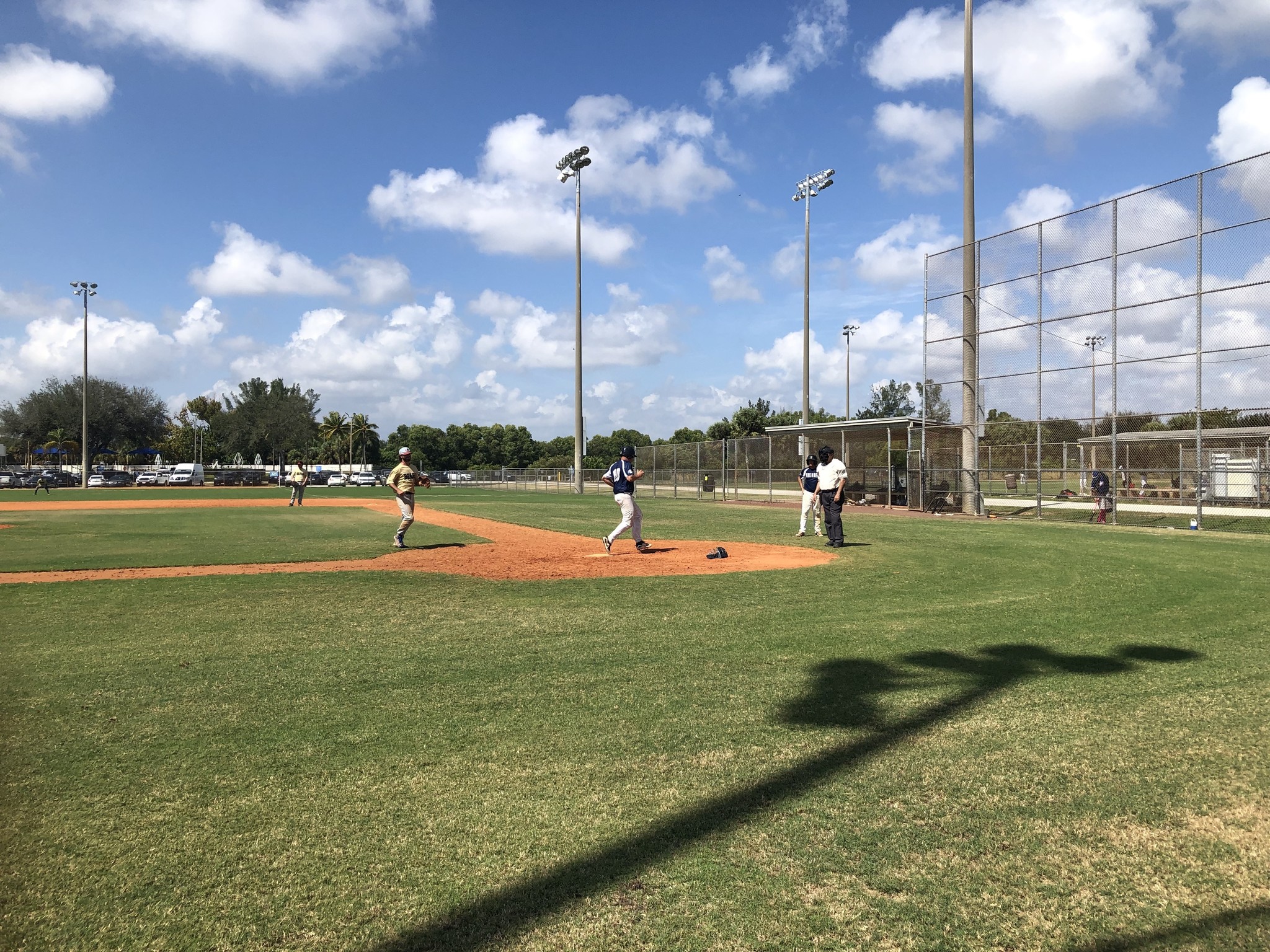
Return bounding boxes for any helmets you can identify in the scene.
[806,455,818,466]
[620,446,637,459]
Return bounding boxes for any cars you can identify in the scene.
[88,475,106,487]
[240,472,262,486]
[268,469,393,487]
[356,472,377,486]
[382,474,390,486]
[418,470,449,483]
[214,472,235,486]
[443,471,471,481]
[327,474,346,487]
[25,476,59,488]
[105,475,133,487]
[16,467,176,488]
[489,470,517,481]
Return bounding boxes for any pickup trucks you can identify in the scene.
[136,472,169,487]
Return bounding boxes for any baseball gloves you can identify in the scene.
[418,476,430,488]
[292,481,298,487]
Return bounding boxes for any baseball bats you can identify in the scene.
[925,492,938,513]
[937,492,951,514]
[932,492,945,514]
[1089,500,1098,522]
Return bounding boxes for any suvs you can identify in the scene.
[0,471,22,489]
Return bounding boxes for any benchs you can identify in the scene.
[1086,487,1197,498]
[845,490,963,505]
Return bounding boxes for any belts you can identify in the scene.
[823,488,839,493]
[629,493,632,495]
[405,492,414,494]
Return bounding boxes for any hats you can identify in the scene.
[41,476,44,478]
[820,445,834,454]
[297,461,303,465]
[399,447,411,455]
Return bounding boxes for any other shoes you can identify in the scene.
[33,494,37,495]
[48,494,50,495]
[288,503,293,506]
[298,504,303,506]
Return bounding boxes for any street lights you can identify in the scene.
[842,325,860,467]
[792,168,836,468]
[69,280,98,489]
[556,146,592,495]
[1084,335,1107,475]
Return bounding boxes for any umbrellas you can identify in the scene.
[127,447,163,454]
[32,447,70,453]
[78,447,120,454]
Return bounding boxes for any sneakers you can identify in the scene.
[394,534,404,547]
[824,541,834,547]
[833,542,843,548]
[815,531,823,536]
[602,536,612,554]
[634,540,652,551]
[795,531,805,536]
[393,541,409,547]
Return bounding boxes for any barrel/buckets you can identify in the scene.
[1190,518,1197,530]
[1004,474,1017,489]
[703,474,714,492]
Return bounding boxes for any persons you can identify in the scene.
[795,455,823,537]
[811,445,849,548]
[287,460,308,507]
[602,446,652,554]
[386,447,430,548]
[1091,471,1110,522]
[1019,466,1026,484]
[1120,464,1148,497]
[33,476,50,495]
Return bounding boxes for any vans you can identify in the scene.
[169,463,205,487]
